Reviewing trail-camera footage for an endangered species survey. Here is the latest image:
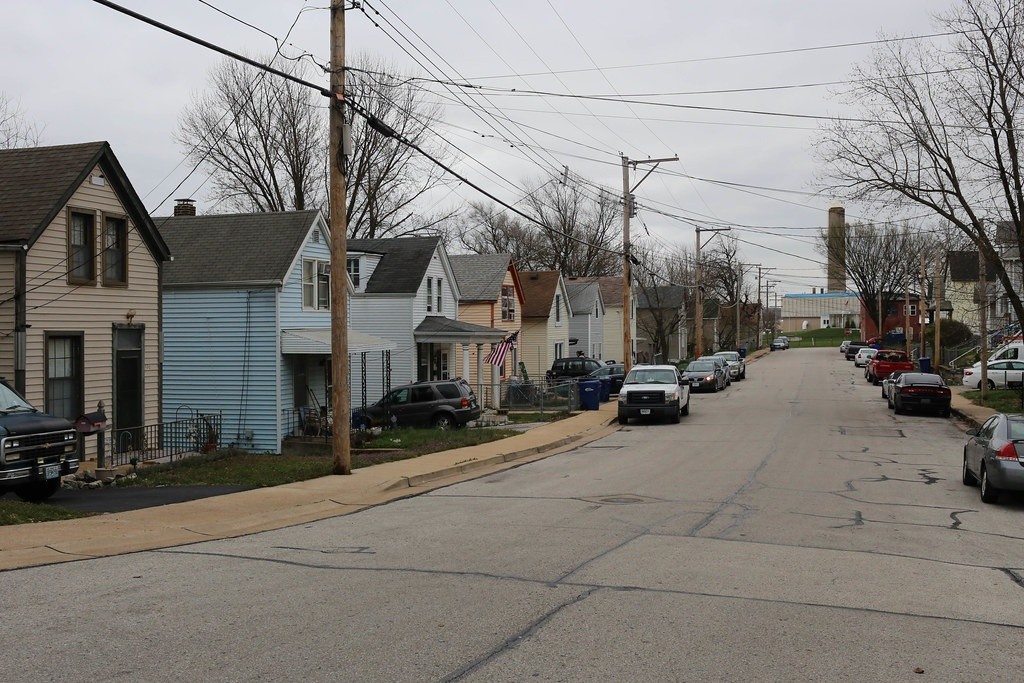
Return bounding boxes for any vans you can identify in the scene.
[548,358,608,385]
[973,341,1024,367]
[778,336,790,349]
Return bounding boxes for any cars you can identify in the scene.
[714,351,746,381]
[353,378,481,430]
[682,360,726,393]
[0,379,79,502]
[698,356,730,386]
[770,339,785,351]
[839,341,878,367]
[887,372,952,415]
[573,363,635,394]
[962,360,1024,391]
[881,370,916,399]
[962,412,1024,504]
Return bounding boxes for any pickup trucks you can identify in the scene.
[617,364,691,425]
[866,350,913,385]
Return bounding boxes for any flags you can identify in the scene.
[483,327,521,366]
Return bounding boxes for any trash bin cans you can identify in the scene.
[738,348,746,359]
[918,357,931,374]
[577,376,611,411]
